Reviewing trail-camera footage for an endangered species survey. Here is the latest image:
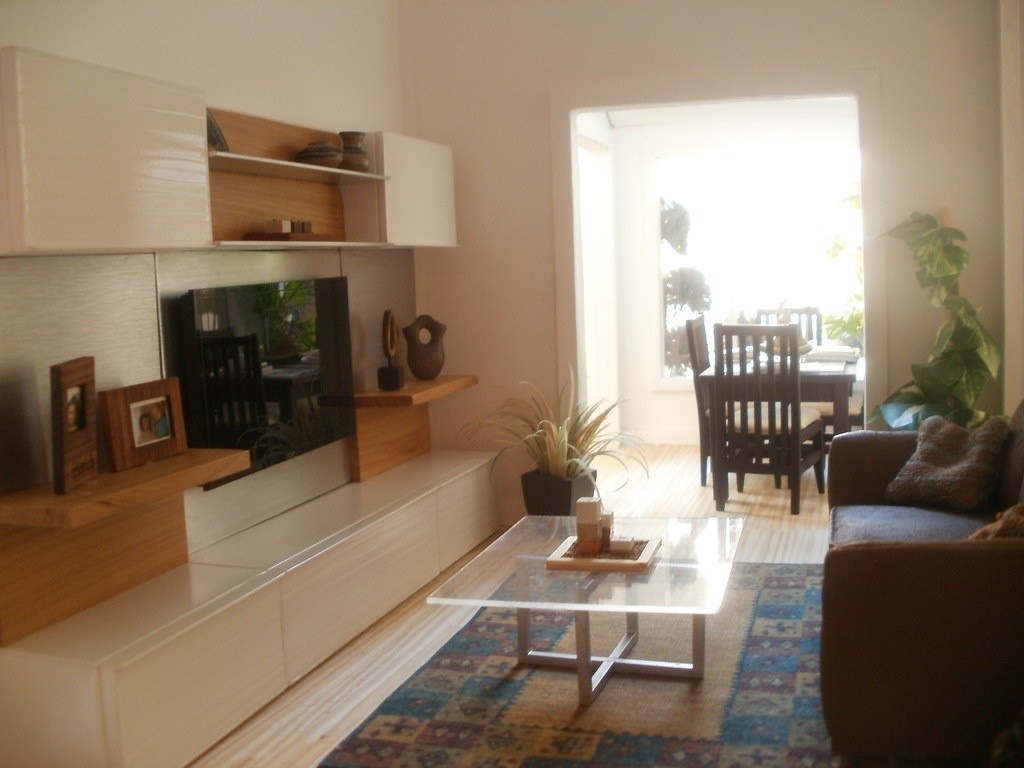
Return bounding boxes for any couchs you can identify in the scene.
[820,399,1024,767]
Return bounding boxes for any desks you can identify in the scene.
[699,362,856,500]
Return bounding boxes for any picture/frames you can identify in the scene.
[97,377,187,474]
[50,356,97,495]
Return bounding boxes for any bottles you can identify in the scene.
[295,139,344,168]
[339,131,370,173]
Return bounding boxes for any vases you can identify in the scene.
[340,132,368,171]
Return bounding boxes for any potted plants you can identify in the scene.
[453,361,651,516]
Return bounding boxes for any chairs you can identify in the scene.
[686,308,866,515]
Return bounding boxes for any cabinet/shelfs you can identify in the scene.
[0,44,211,256]
[205,106,395,249]
[339,131,457,248]
[1,374,502,768]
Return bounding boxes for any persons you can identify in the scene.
[135,403,169,444]
[65,395,82,432]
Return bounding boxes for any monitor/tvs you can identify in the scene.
[177,276,356,492]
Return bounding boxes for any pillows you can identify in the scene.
[970,502,1024,539]
[884,415,1012,515]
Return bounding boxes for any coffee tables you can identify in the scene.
[425,516,749,706]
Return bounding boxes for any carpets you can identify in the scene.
[315,563,849,768]
